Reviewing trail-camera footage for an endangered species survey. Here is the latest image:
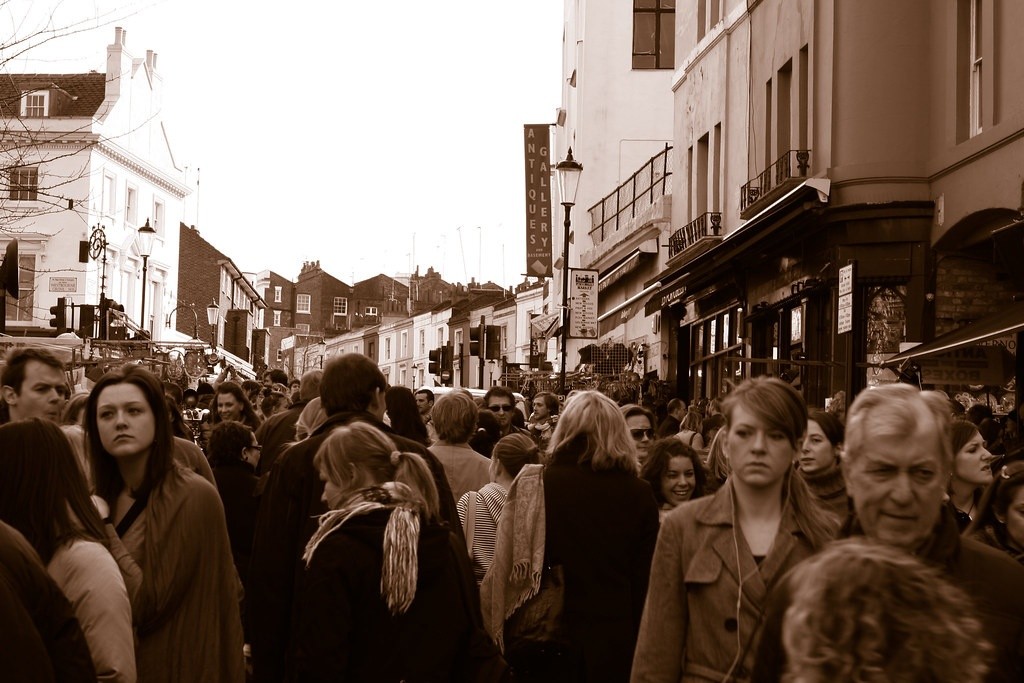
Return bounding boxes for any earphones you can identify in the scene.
[791,449,798,463]
[724,441,729,458]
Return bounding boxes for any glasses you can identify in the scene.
[487,405,513,412]
[630,429,655,440]
[242,444,262,451]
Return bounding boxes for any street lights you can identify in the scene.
[411,364,418,394]
[555,146,584,396]
[317,338,327,370]
[207,298,221,348]
[137,217,157,329]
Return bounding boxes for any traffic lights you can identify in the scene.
[469,324,483,358]
[49,297,67,334]
[428,348,441,377]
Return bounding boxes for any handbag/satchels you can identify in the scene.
[500,564,566,663]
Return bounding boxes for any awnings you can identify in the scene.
[873,291,1024,374]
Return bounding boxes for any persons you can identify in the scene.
[0,346,1024,683]
